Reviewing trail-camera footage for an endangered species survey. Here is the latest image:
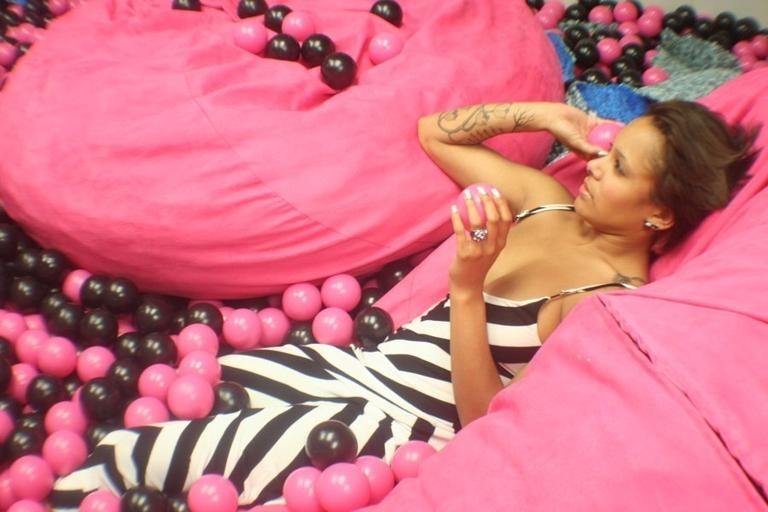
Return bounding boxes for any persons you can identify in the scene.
[0,99,759,508]
